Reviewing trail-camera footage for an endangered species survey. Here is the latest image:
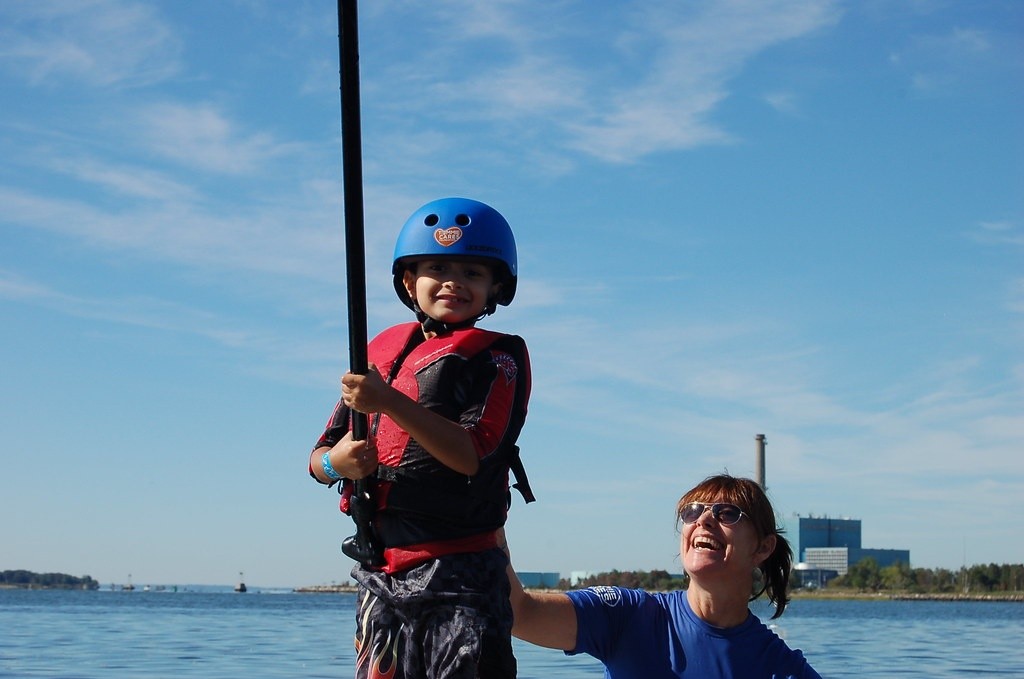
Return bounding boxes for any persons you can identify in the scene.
[307,198,533,679]
[495,473,822,679]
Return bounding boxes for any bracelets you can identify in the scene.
[500,540,508,551]
[321,450,341,479]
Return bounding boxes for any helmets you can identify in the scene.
[393,197,518,313]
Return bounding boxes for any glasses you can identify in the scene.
[678,502,756,526]
